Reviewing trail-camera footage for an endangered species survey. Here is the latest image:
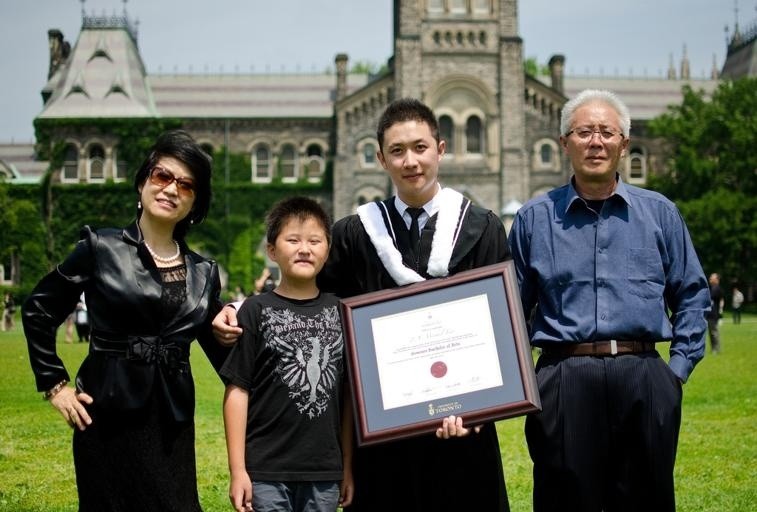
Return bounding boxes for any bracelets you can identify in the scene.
[40,378,68,402]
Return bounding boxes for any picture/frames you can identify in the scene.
[339,258,543,447]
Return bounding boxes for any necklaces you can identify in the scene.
[142,238,183,264]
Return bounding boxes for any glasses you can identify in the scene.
[148,167,195,196]
[566,127,625,138]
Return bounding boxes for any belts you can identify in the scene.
[570,342,651,356]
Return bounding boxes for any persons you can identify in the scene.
[211,95,515,512]
[218,195,357,512]
[0,269,91,345]
[703,271,726,355]
[17,126,232,512]
[505,90,712,511]
[730,287,744,325]
[228,268,279,303]
